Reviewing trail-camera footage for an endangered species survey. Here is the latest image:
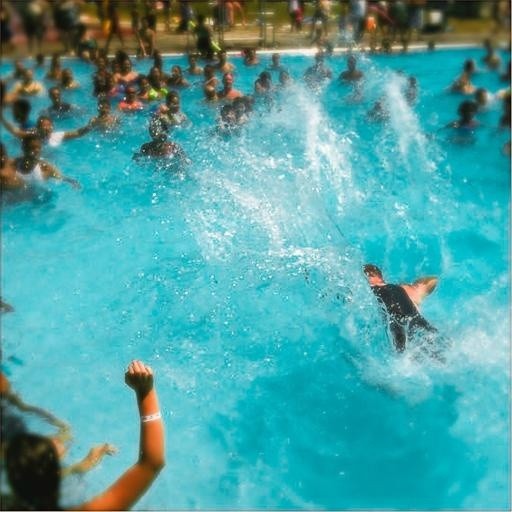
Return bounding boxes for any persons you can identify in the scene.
[1,135,81,196]
[305,262,441,373]
[1,358,167,511]
[134,116,191,166]
[0,1,511,150]
